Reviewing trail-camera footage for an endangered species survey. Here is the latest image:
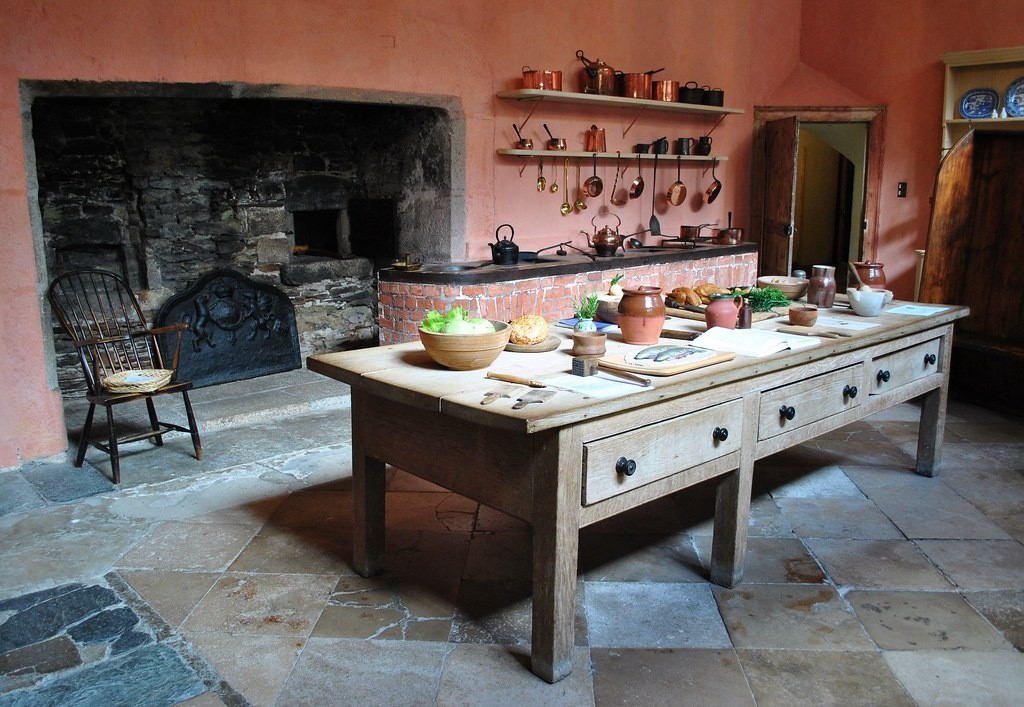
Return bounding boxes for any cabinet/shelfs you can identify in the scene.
[496,89,746,160]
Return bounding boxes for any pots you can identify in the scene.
[419,123,744,272]
[519,50,724,108]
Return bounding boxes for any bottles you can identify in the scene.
[1000,106,1007,119]
[849,261,886,290]
[618,284,666,345]
[807,264,836,308]
[991,109,998,119]
[793,270,806,279]
[739,298,752,329]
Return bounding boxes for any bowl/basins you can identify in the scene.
[636,143,651,154]
[418,317,513,371]
[758,276,809,300]
[391,261,422,271]
[848,288,893,317]
[587,292,620,325]
[789,306,818,327]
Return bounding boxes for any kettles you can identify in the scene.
[488,224,518,265]
[586,124,607,152]
[580,213,623,257]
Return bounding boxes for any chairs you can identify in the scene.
[46,269,203,486]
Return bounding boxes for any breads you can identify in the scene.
[509,315,549,345]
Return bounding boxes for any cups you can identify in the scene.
[571,332,607,358]
[677,138,695,156]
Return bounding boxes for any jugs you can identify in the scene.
[698,136,712,156]
[704,292,743,331]
[652,138,668,154]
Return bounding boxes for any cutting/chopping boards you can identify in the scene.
[596,343,736,377]
[662,302,817,326]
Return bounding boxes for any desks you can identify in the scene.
[307,295,970,684]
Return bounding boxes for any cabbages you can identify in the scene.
[421,305,497,335]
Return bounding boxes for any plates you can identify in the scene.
[959,87,1000,119]
[505,335,561,352]
[1004,76,1024,118]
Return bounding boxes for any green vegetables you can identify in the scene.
[735,287,792,312]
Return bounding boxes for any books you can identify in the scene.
[554,318,619,333]
[690,325,820,357]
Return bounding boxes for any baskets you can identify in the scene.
[105,369,173,393]
